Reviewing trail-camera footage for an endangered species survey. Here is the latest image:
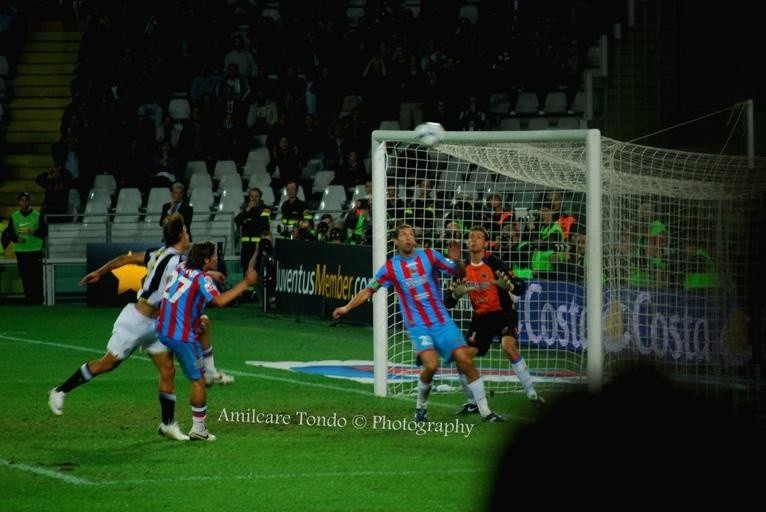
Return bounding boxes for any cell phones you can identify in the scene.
[175,200,181,203]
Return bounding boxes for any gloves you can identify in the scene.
[448,277,478,300]
[488,270,515,293]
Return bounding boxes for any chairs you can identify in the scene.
[48,1,614,256]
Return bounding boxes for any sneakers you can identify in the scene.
[456,403,480,418]
[23,298,44,305]
[46,385,66,417]
[528,395,547,409]
[188,430,217,443]
[157,419,190,442]
[480,412,508,423]
[415,406,427,422]
[204,371,237,389]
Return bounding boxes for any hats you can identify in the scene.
[18,192,30,199]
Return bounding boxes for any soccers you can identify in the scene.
[415,121,443,145]
